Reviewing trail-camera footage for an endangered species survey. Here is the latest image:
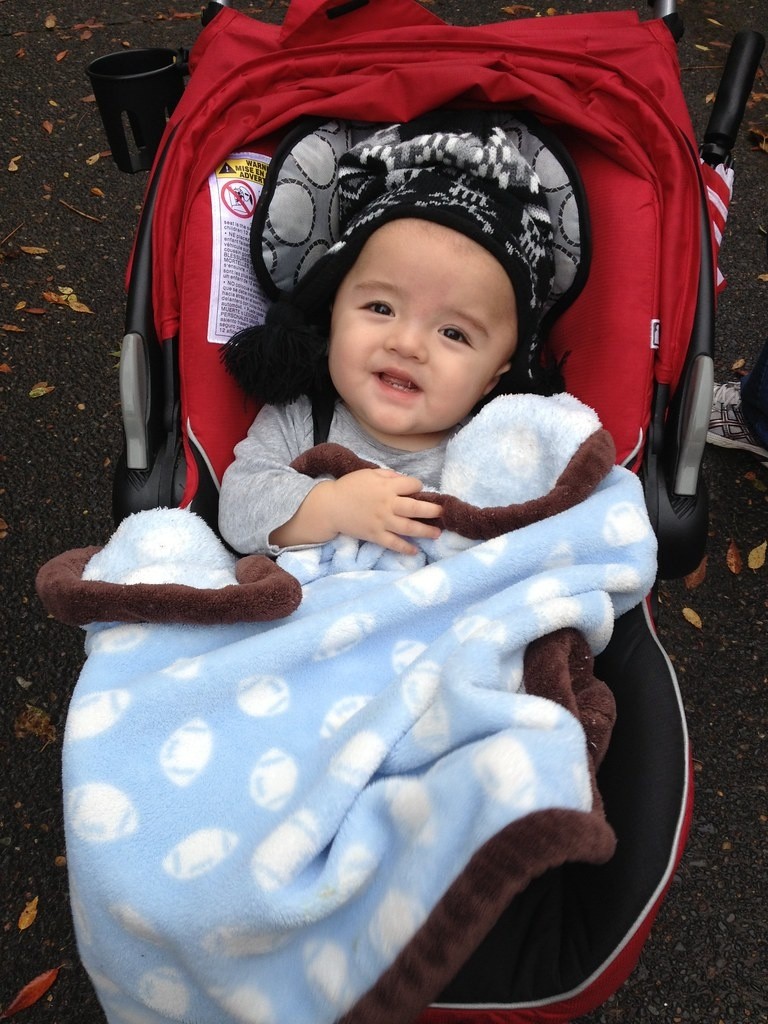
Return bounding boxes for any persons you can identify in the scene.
[217,111,555,567]
[705,335,768,458]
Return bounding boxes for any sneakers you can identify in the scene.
[705,381,768,458]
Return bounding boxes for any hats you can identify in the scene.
[217,119,572,407]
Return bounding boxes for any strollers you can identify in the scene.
[84,0,768,1024]
